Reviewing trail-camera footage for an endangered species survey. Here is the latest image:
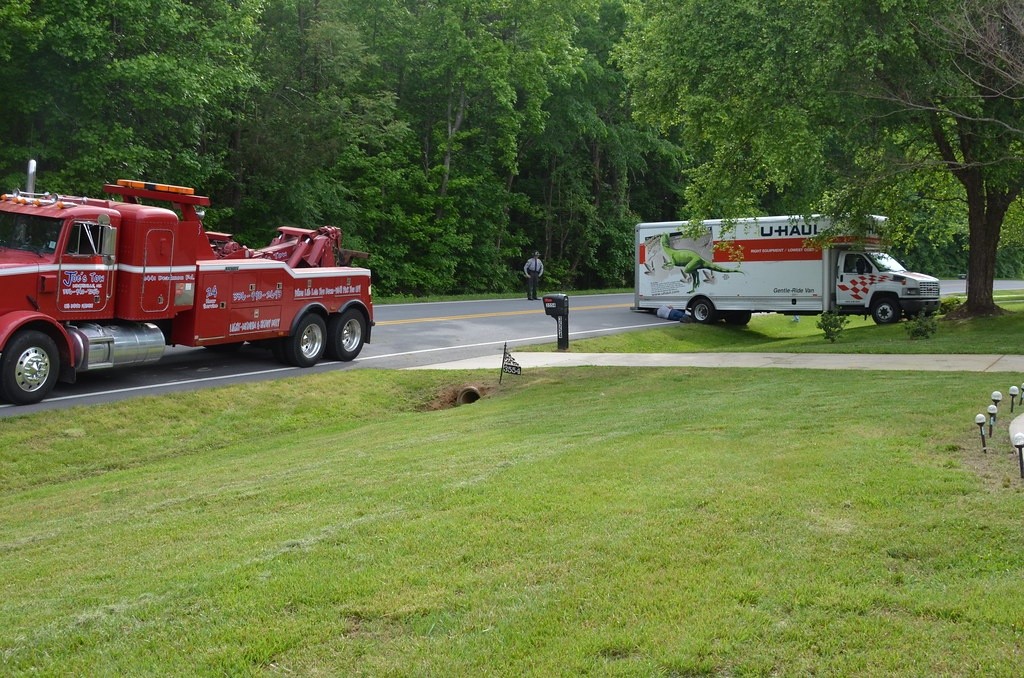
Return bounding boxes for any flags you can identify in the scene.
[502,348,521,375]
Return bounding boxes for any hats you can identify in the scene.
[532,251,541,255]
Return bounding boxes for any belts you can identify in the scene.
[531,271,539,273]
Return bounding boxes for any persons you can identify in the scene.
[524,250,544,301]
[652,304,693,323]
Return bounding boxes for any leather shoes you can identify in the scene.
[528,297,541,300]
[679,315,691,323]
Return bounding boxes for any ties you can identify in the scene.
[535,258,537,270]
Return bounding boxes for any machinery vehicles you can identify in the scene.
[0,158,376,406]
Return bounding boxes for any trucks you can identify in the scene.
[629,212,941,325]
